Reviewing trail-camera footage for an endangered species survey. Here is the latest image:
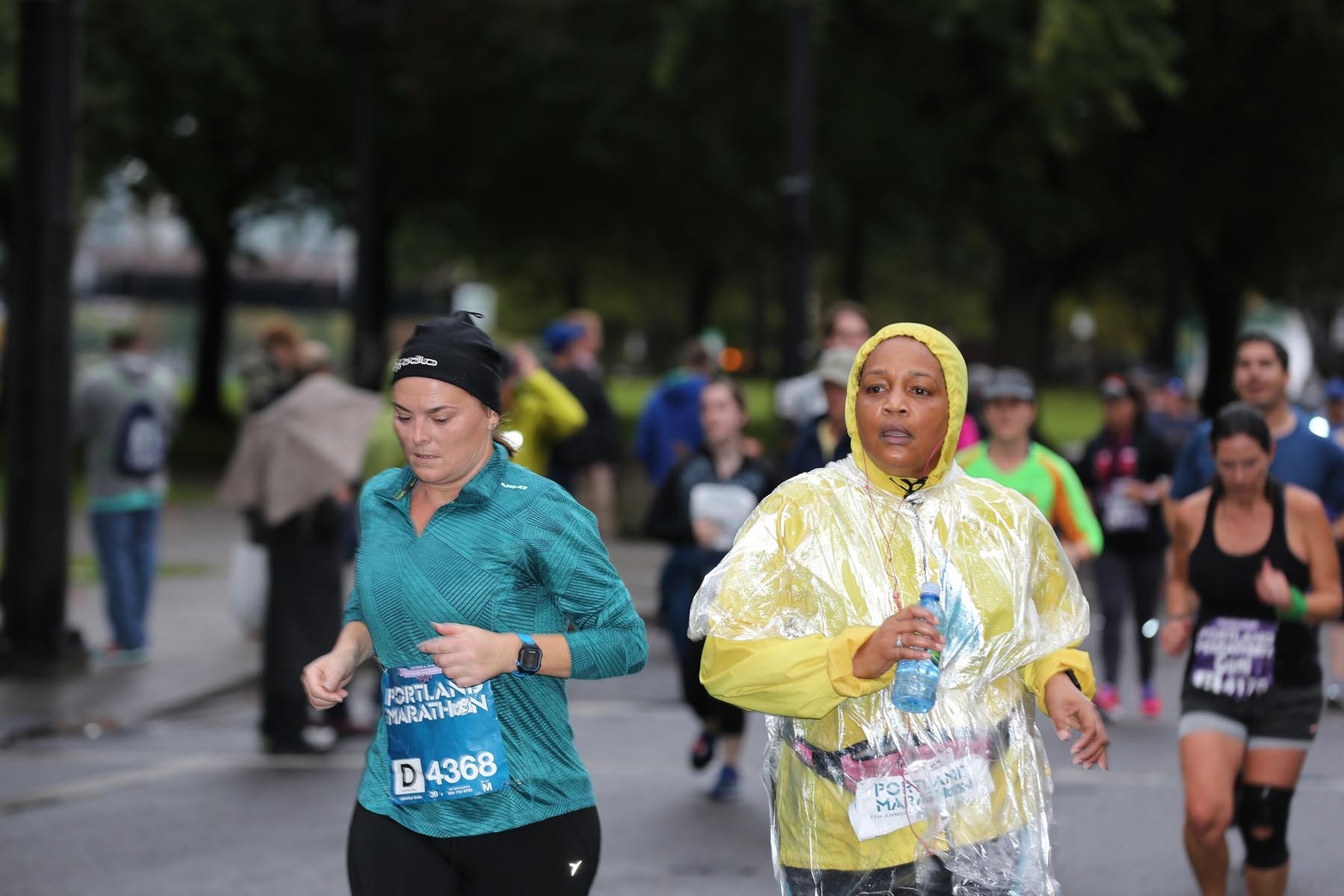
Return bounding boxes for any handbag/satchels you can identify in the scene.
[228,542,270,644]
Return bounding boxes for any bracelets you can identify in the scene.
[1067,668,1083,691]
[1273,585,1310,625]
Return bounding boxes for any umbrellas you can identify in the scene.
[209,371,384,526]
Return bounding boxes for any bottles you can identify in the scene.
[891,581,949,714]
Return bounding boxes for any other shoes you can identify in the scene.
[1326,679,1344,711]
[706,766,738,802]
[264,727,338,754]
[691,728,717,769]
[1139,681,1165,717]
[1092,682,1123,723]
[322,714,372,737]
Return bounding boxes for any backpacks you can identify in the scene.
[111,399,163,480]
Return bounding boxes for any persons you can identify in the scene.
[686,322,1112,896]
[240,284,1344,806]
[71,313,175,653]
[299,310,650,896]
[1161,405,1344,896]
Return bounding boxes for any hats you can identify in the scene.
[543,320,587,346]
[811,346,853,387]
[1100,375,1133,401]
[982,367,1034,401]
[389,311,505,417]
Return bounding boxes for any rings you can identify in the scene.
[897,633,905,648]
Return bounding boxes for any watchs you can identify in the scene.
[513,631,542,679]
